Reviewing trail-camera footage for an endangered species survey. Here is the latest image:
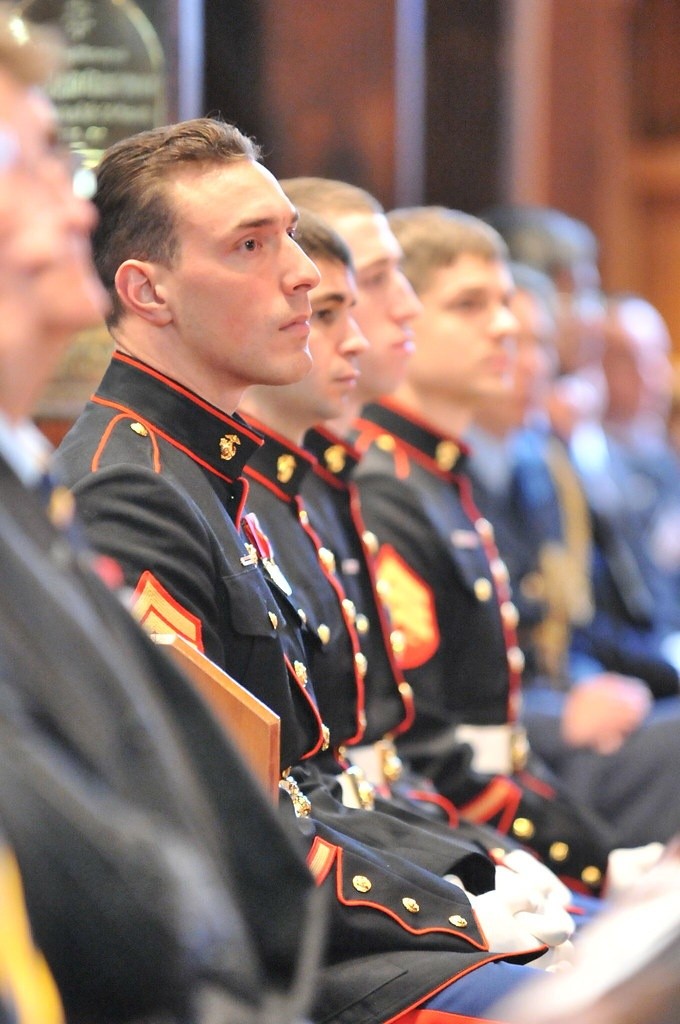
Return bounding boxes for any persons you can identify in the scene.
[58,119,586,955]
[0,1,679,1024]
